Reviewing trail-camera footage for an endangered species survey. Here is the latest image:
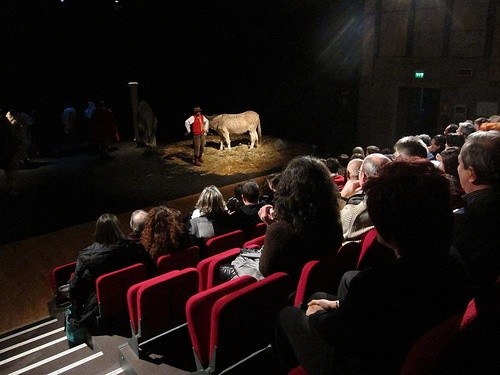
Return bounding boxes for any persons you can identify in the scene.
[67,115,500,375]
[185,107,209,166]
[61,98,154,150]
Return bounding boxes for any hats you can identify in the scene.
[193,107,202,112]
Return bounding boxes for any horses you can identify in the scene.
[206,110,262,152]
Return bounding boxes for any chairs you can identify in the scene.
[51,222,500,375]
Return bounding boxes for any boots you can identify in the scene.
[199,152,204,162]
[194,155,201,165]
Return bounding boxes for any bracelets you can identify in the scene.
[335,300,339,308]
